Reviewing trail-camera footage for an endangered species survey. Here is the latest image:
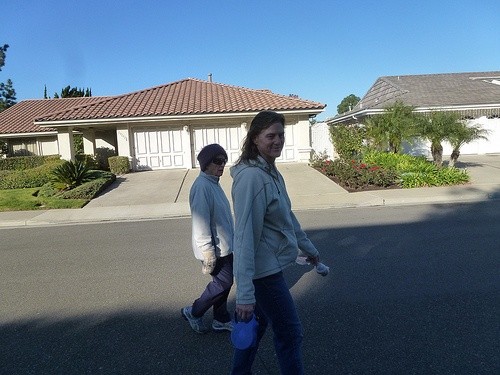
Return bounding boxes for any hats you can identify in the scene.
[197,144,228,172]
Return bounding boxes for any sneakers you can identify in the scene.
[211,318,233,331]
[181,306,207,335]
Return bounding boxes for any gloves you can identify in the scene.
[202,251,216,275]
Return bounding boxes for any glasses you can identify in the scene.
[212,158,227,165]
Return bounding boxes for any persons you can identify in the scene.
[178,143,236,335]
[230,110,321,375]
